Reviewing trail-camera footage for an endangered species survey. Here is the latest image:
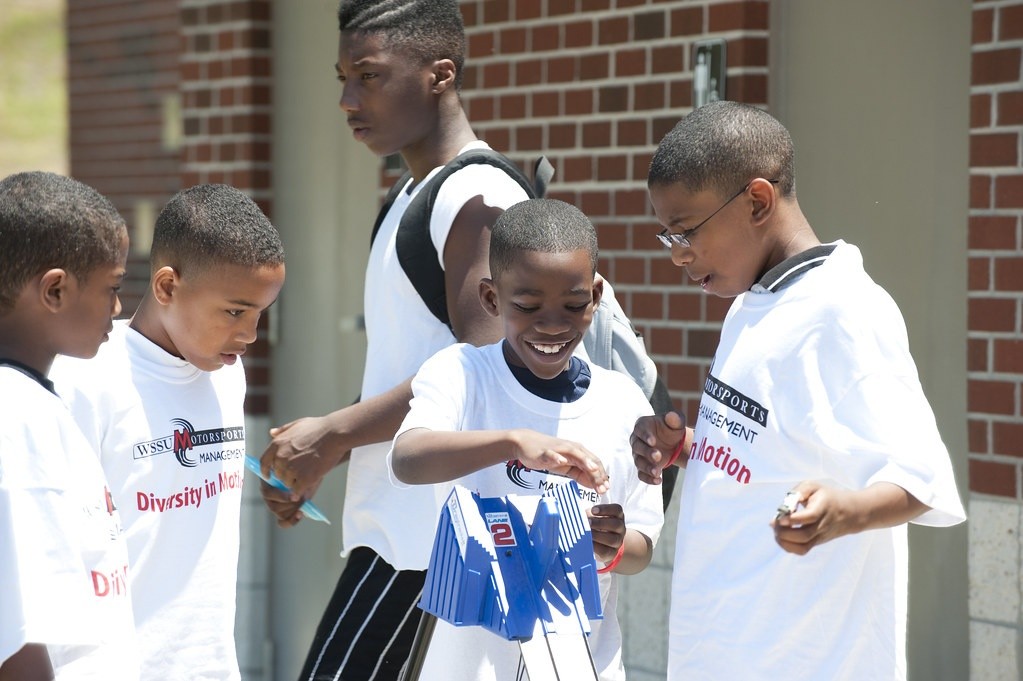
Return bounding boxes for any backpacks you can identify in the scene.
[369,149,680,512]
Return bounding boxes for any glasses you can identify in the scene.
[655,179,781,248]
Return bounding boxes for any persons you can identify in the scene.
[258,1,539,681]
[0,171,137,681]
[385,197,665,681]
[47,182,286,681]
[627,99,968,681]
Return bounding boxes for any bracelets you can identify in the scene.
[596,542,626,575]
[666,431,687,468]
